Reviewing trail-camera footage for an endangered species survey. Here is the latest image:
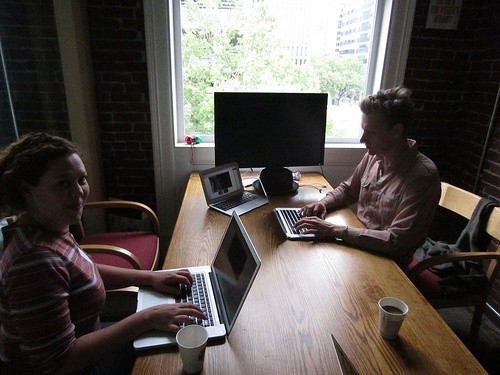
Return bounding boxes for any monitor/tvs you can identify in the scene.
[213,91,328,194]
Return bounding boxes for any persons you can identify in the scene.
[0,131,208,375]
[292,86,441,271]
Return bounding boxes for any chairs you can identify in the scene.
[409,181,500,342]
[75,201,161,271]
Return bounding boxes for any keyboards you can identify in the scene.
[272,207,317,239]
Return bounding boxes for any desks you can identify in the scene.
[131,171,488,375]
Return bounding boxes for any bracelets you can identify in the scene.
[342,227,348,239]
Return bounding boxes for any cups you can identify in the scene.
[378,297,409,341]
[175,325,209,373]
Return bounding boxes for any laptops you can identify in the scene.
[198,160,268,217]
[131,208,263,352]
